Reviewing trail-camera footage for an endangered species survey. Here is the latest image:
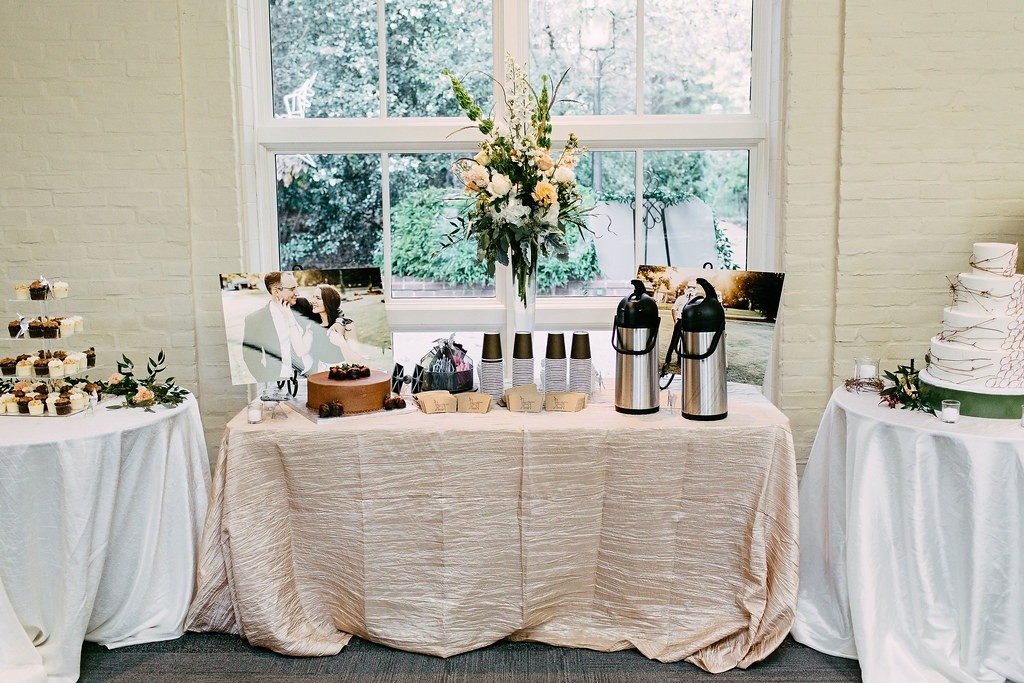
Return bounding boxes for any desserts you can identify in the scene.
[8,316,83,339]
[14,278,68,300]
[0,381,102,416]
[0,347,96,378]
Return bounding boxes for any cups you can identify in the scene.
[544,332,567,408]
[512,331,534,388]
[481,331,503,402]
[569,331,591,403]
[853,357,881,391]
[942,401,962,424]
[1021,405,1024,427]
[248,401,263,423]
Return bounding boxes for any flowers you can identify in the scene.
[102,348,191,413]
[440,53,617,309]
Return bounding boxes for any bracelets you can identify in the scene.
[289,322,299,331]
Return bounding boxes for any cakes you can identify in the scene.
[926,242,1024,392]
[306,363,406,417]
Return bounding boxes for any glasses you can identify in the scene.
[279,285,296,291]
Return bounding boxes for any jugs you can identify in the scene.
[675,278,728,421]
[611,279,660,415]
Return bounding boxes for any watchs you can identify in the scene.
[336,318,346,325]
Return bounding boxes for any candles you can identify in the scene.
[248,408,261,421]
[944,406,958,422]
[860,364,875,381]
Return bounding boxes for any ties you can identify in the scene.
[290,342,304,374]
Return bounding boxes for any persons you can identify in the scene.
[242,272,352,381]
[274,284,360,373]
[671,281,697,371]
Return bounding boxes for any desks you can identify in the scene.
[184,390,801,674]
[790,379,1023,683]
[0,386,211,682]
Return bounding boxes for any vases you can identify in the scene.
[512,239,537,331]
